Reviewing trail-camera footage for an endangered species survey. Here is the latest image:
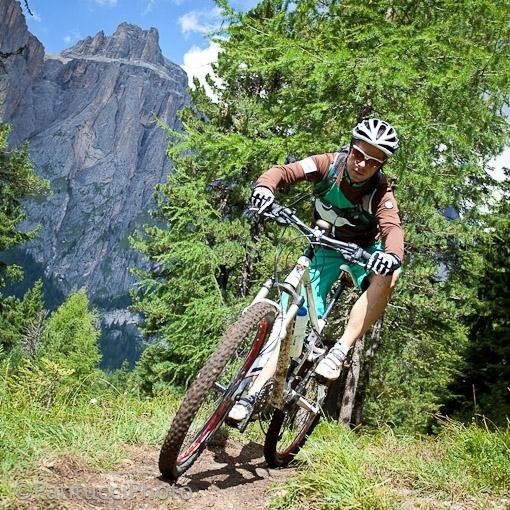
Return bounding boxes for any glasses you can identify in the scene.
[350,141,388,169]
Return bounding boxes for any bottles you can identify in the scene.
[289,306,308,357]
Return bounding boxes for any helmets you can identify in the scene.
[351,118,400,158]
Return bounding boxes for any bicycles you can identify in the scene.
[158,202,372,480]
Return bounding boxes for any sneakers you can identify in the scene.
[314,339,355,384]
[227,393,262,422]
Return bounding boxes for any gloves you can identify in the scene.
[250,185,276,214]
[366,250,402,277]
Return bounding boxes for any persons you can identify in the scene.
[226,117,405,423]
